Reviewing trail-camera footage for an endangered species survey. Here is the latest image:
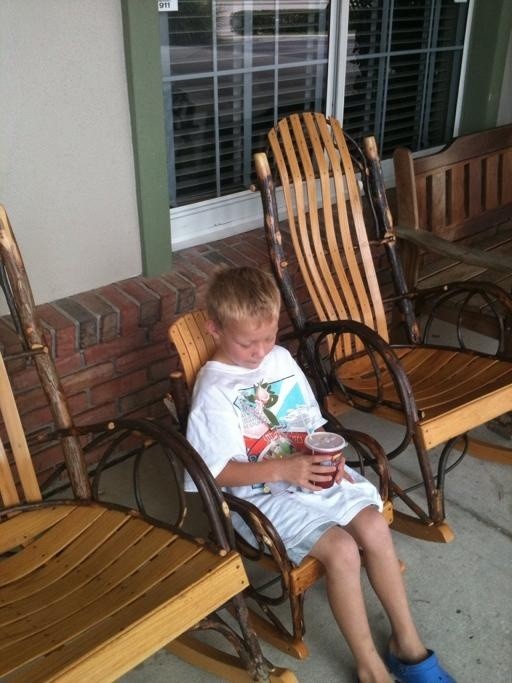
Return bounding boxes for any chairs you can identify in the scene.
[163,307,404,660]
[0,205,298,683]
[249,111,512,543]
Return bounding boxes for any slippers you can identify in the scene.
[384,645,455,683]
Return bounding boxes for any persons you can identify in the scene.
[183,264,457,683]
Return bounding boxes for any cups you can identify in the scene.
[304,432,345,489]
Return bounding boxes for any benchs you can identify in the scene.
[394,121,512,339]
